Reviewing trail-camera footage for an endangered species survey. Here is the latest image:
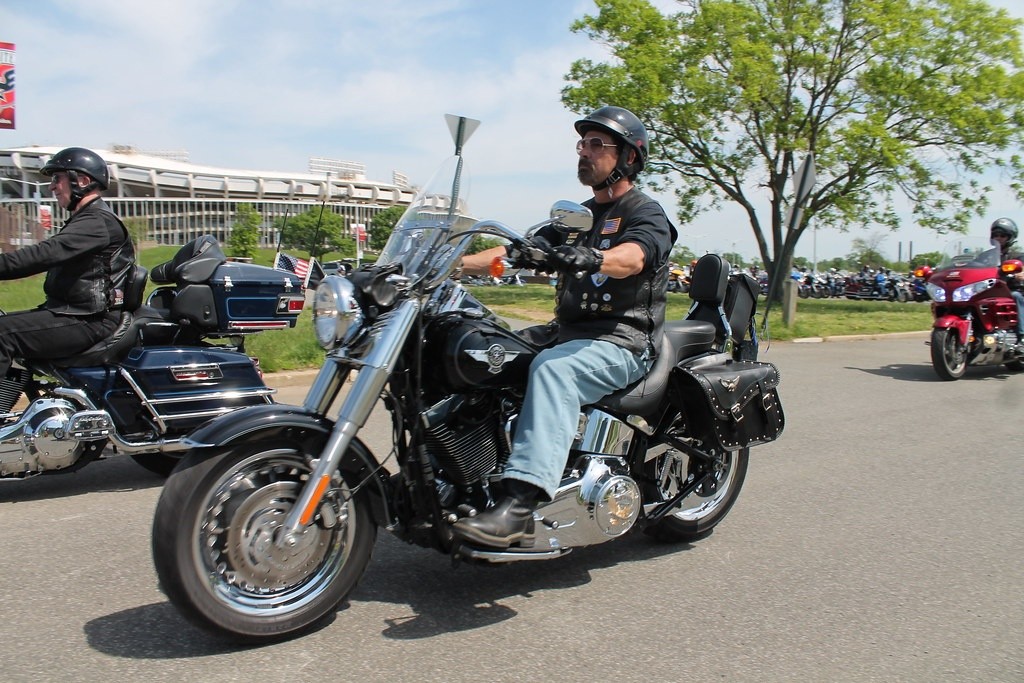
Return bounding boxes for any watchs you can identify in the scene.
[590,247,604,272]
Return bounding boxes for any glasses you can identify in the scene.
[993,233,1007,238]
[51,174,84,183]
[576,137,620,155]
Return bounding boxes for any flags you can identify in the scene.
[274,253,309,288]
[303,257,325,291]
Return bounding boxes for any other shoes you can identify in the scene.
[1014,338,1024,353]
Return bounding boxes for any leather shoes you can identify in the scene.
[454,482,539,548]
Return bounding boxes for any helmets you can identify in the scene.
[39,147,109,191]
[667,258,890,277]
[990,218,1018,247]
[574,106,649,174]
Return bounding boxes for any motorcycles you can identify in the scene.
[154,112,785,643]
[924,241,1023,380]
[667,259,932,302]
[0,238,309,481]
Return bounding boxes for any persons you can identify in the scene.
[337,258,352,272]
[974,217,1024,353]
[455,106,678,547]
[0,147,136,381]
[669,260,926,296]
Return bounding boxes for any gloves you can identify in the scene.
[538,243,603,274]
